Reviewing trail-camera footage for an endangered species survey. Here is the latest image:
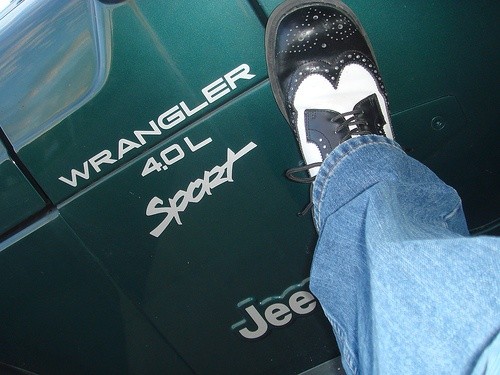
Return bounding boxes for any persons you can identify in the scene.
[264,0,500,375]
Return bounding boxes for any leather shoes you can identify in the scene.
[265,1,397,194]
